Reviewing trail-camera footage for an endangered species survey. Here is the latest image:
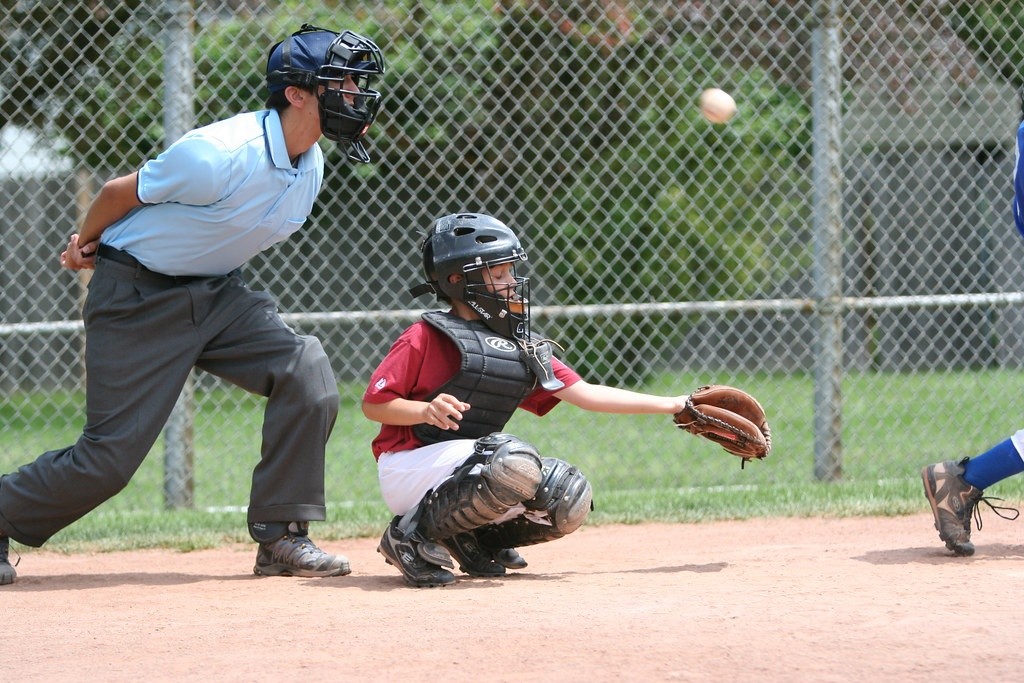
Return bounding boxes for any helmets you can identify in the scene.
[409,213,531,342]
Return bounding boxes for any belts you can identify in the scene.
[96,243,208,284]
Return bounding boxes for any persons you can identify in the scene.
[1,21,386,577]
[920,116,1024,554]
[361,211,770,587]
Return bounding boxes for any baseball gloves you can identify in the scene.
[672,384,773,463]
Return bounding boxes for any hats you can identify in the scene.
[267,31,375,93]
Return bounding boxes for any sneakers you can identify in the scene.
[251,520,350,578]
[445,532,506,578]
[377,515,457,587]
[0,530,20,584]
[922,457,1019,556]
